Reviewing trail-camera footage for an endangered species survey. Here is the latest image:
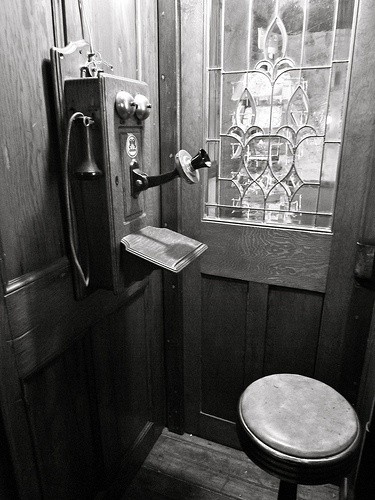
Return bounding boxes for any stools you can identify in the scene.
[236,373,362,498]
